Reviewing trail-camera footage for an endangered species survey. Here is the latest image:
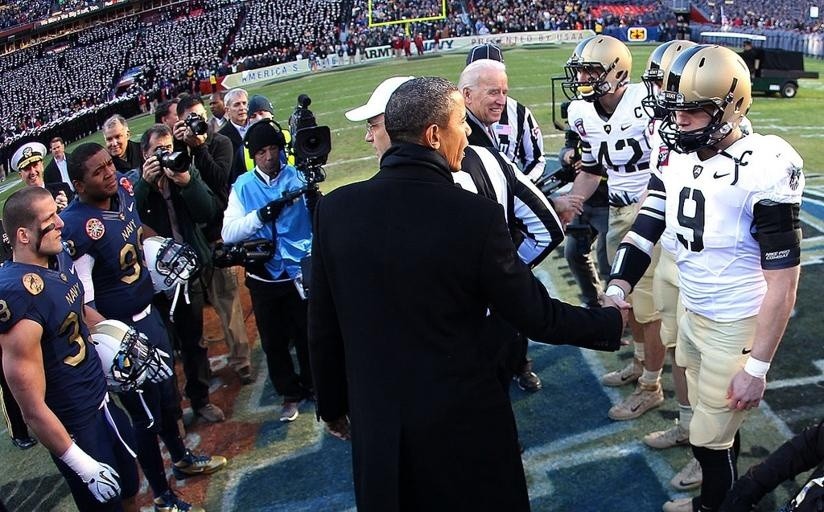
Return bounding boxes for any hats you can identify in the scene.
[344,74,416,123]
[244,119,287,159]
[466,43,504,64]
[247,94,275,118]
[9,141,48,172]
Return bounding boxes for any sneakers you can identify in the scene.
[513,370,543,393]
[663,498,714,512]
[608,379,663,420]
[670,455,704,491]
[601,360,643,387]
[279,401,300,423]
[193,402,226,424]
[152,491,207,512]
[236,366,252,385]
[173,451,227,481]
[642,422,691,450]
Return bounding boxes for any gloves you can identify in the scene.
[261,198,286,223]
[57,442,122,504]
[305,191,324,214]
[143,345,174,383]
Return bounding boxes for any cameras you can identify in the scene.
[178,112,210,137]
[149,148,190,175]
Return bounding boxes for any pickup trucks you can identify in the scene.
[699,30,818,100]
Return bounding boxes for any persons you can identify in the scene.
[154,98,177,132]
[346,74,565,271]
[550,0,600,30]
[336,40,346,66]
[221,118,320,423]
[435,31,440,53]
[6,142,75,215]
[172,95,253,387]
[308,48,317,73]
[309,1,367,44]
[290,93,317,148]
[738,39,755,76]
[558,35,667,421]
[346,37,357,65]
[563,97,633,311]
[358,36,368,61]
[318,43,328,70]
[448,57,585,215]
[403,36,412,56]
[721,404,824,512]
[129,123,225,424]
[653,44,804,512]
[219,0,309,76]
[693,0,823,34]
[219,89,254,185]
[414,32,425,55]
[102,91,138,116]
[459,45,545,392]
[0,0,63,139]
[138,0,219,114]
[54,142,226,510]
[449,1,550,35]
[65,1,137,90]
[392,34,403,58]
[240,97,294,173]
[100,115,148,173]
[42,138,82,201]
[601,1,694,37]
[368,0,391,47]
[1,187,143,511]
[600,39,753,450]
[393,0,451,34]
[208,92,228,128]
[57,90,101,137]
[305,78,630,510]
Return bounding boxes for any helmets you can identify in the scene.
[657,46,753,155]
[641,39,699,121]
[142,234,200,294]
[90,318,162,393]
[561,34,634,104]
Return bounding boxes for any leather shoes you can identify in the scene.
[13,436,39,450]
